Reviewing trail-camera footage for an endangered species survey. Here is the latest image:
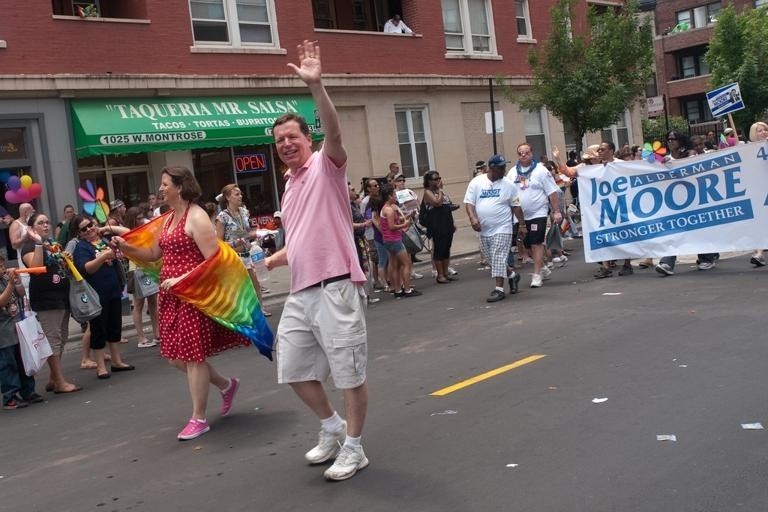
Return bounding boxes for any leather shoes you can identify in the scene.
[46,380,68,392]
[53,384,83,394]
[111,364,135,372]
[97,368,111,379]
[447,274,459,280]
[437,278,452,284]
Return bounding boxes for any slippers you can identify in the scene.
[639,261,655,270]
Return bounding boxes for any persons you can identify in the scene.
[0,164,286,441]
[246,39,370,480]
[729,89,740,104]
[384,14,416,36]
[347,122,768,302]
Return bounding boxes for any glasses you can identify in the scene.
[78,222,94,232]
[430,177,441,181]
[349,188,355,192]
[371,184,378,188]
[597,148,606,152]
[517,151,531,156]
[33,220,48,226]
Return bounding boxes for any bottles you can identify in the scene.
[249,241,271,284]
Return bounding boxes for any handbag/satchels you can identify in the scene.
[546,221,564,250]
[69,279,102,324]
[133,270,160,300]
[14,316,54,377]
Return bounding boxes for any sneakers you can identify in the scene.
[410,272,424,280]
[262,309,272,317]
[618,266,633,276]
[305,420,347,464]
[367,297,380,303]
[562,250,573,256]
[592,267,613,279]
[447,268,458,275]
[430,271,438,278]
[219,375,241,417]
[177,418,210,440]
[540,266,552,281]
[560,255,569,264]
[3,396,29,410]
[394,289,405,298]
[137,341,156,349]
[523,257,534,264]
[261,287,270,293]
[750,254,766,266]
[405,288,423,297]
[530,273,544,288]
[323,443,369,481]
[372,282,384,289]
[383,284,393,293]
[104,353,112,362]
[549,258,564,268]
[80,360,97,369]
[655,264,675,276]
[153,338,161,343]
[573,233,582,237]
[508,271,521,294]
[120,338,129,343]
[698,260,716,270]
[21,392,43,404]
[487,289,505,302]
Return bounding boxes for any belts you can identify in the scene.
[305,273,351,288]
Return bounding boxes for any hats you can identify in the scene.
[582,144,600,160]
[476,160,486,169]
[109,199,124,211]
[723,127,735,136]
[394,173,406,180]
[273,210,282,219]
[488,153,511,168]
